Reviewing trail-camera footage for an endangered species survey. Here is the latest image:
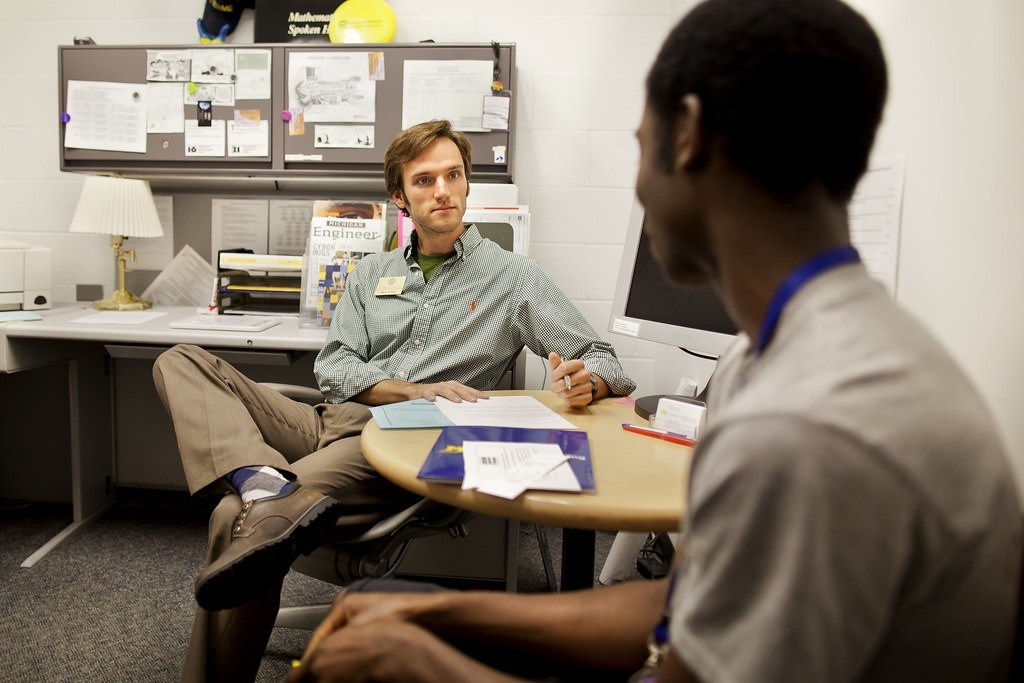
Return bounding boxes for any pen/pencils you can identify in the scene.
[621,423,701,448]
[560,355,572,391]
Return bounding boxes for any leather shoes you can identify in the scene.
[195,483,344,612]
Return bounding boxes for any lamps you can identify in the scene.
[70,176,164,312]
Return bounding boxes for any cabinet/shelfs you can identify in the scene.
[58,43,517,190]
[216,248,303,315]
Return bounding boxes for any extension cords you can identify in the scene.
[636,558,668,580]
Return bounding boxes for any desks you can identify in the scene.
[361,389,688,592]
[0,303,526,594]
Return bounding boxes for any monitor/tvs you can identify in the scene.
[606,189,741,422]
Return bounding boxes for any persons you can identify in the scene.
[288,0,1024,683]
[153,120,636,682]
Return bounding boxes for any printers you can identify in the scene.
[0,243,51,310]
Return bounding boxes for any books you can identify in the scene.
[299,216,385,330]
[312,199,388,217]
